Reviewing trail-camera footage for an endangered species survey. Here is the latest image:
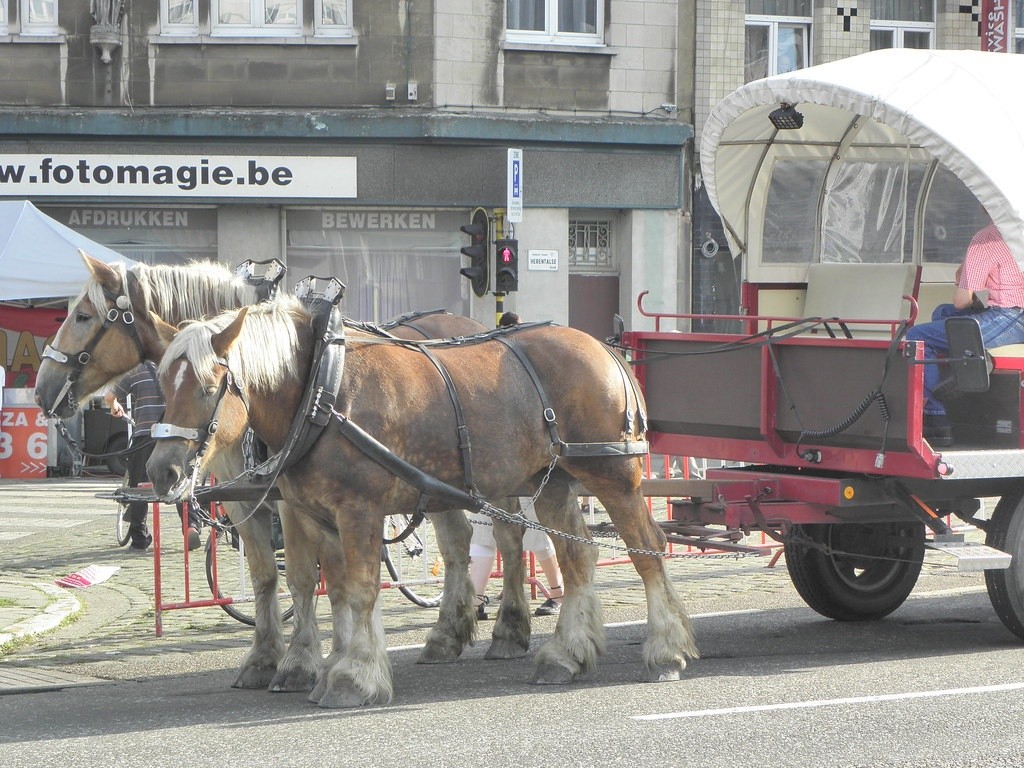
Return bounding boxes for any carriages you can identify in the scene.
[32,47,1024,710]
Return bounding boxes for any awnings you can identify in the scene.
[0,201,143,309]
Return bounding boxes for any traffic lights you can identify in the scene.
[459,205,491,298]
[496,238,519,292]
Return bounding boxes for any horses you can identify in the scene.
[37,248,700,708]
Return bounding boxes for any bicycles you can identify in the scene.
[200,508,449,627]
[113,411,214,547]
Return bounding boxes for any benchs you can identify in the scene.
[914,286,1024,449]
[793,262,923,340]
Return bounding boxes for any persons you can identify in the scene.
[906,202,1024,447]
[104,360,201,550]
[499,311,523,328]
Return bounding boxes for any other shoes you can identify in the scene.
[188,527,201,549]
[923,425,953,448]
[535,599,562,614]
[931,361,993,402]
